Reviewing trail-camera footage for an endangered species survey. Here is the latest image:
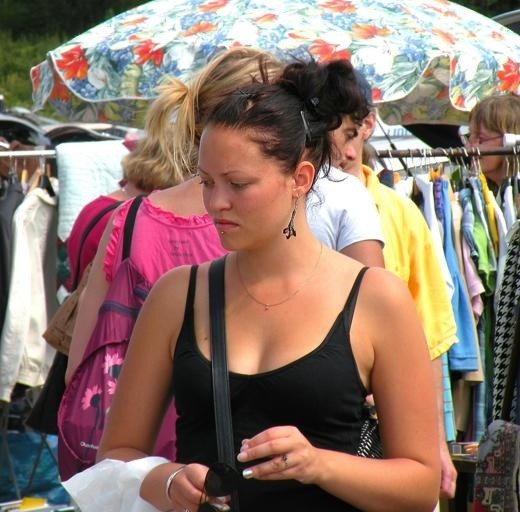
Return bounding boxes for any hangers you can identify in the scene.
[379,146,520,201]
[0,152,56,197]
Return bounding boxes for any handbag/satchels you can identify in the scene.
[42,195,177,482]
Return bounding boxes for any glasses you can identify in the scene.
[468,134,502,144]
[196,462,242,511]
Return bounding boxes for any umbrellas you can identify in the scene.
[30,0,520,126]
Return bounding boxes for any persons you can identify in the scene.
[464,94,520,217]
